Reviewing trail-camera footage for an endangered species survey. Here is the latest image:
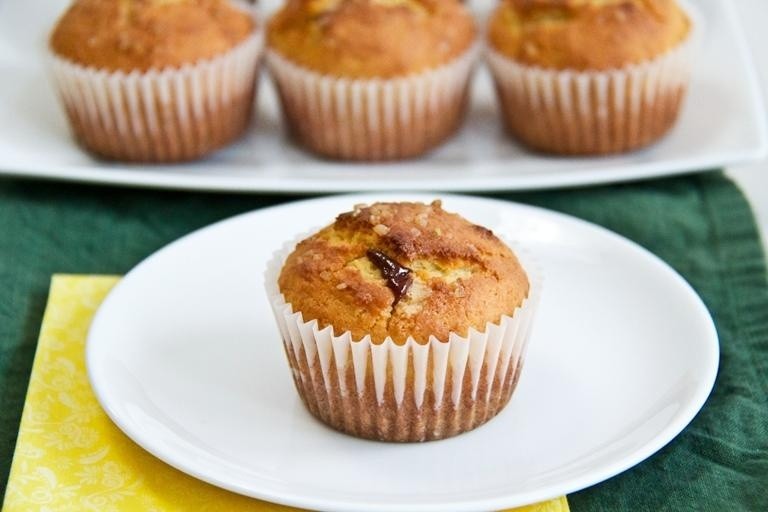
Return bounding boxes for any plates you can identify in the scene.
[85,193,720,511]
[0,0,768,194]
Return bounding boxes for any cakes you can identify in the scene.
[44,0,262,165]
[274,198,532,445]
[261,0,481,163]
[481,0,697,155]
